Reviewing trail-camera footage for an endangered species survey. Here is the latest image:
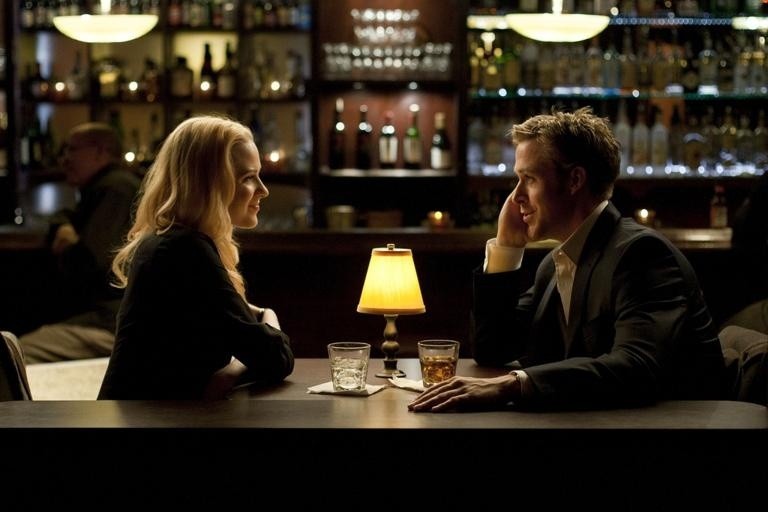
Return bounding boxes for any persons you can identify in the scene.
[93,113,295,402]
[408,108,723,412]
[15,122,144,366]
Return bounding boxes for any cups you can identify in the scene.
[322,196,451,234]
[418,338,462,389]
[327,341,371,395]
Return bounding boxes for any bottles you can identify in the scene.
[323,90,457,172]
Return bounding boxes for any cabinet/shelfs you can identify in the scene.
[2,2,768,230]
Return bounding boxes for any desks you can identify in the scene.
[231,345,525,402]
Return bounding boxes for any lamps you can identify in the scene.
[358,242,432,378]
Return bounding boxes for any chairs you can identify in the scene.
[1,327,32,400]
